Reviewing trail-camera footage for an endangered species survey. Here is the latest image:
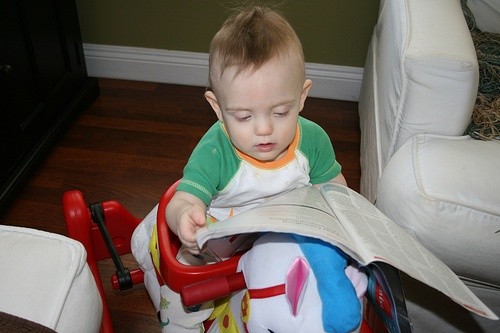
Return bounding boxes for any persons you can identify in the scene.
[155,3,349,333]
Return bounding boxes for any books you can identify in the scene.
[194,181,500,326]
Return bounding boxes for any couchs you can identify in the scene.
[359,0,500,333]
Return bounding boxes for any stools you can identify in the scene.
[0,225,104,333]
